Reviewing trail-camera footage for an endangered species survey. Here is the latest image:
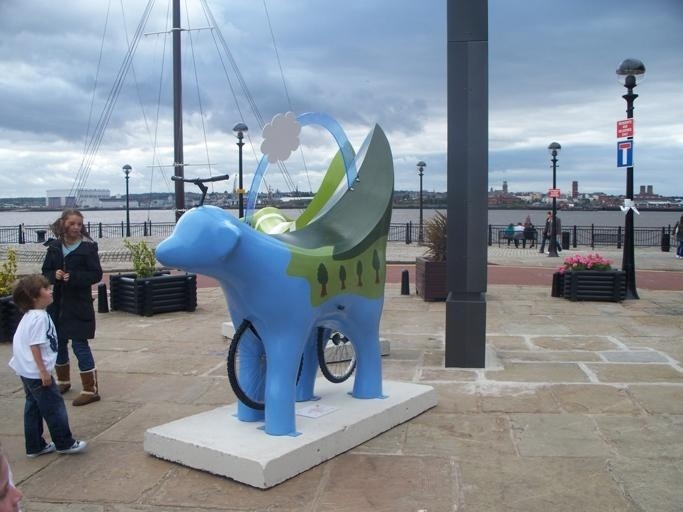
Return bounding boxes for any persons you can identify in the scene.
[8,275,87,459]
[504,211,563,254]
[0,451,23,512]
[672,214,683,258]
[39,207,102,407]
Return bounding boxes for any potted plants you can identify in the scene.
[414,211,448,301]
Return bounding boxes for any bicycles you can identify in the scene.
[222,310,358,406]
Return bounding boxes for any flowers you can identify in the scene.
[555,251,615,277]
[0,248,19,297]
[118,236,157,277]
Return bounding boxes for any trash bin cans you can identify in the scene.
[662,234,670,251]
[562,232,569,249]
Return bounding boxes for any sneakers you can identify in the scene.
[56,439,86,454]
[676,255,683,259]
[26,442,56,457]
[558,243,562,251]
[538,248,545,253]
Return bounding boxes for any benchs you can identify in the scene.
[498,230,539,249]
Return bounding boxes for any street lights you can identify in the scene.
[616,55,644,302]
[548,142,560,258]
[231,123,249,218]
[416,161,425,245]
[123,164,135,239]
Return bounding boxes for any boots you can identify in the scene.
[72,368,101,407]
[54,363,71,394]
